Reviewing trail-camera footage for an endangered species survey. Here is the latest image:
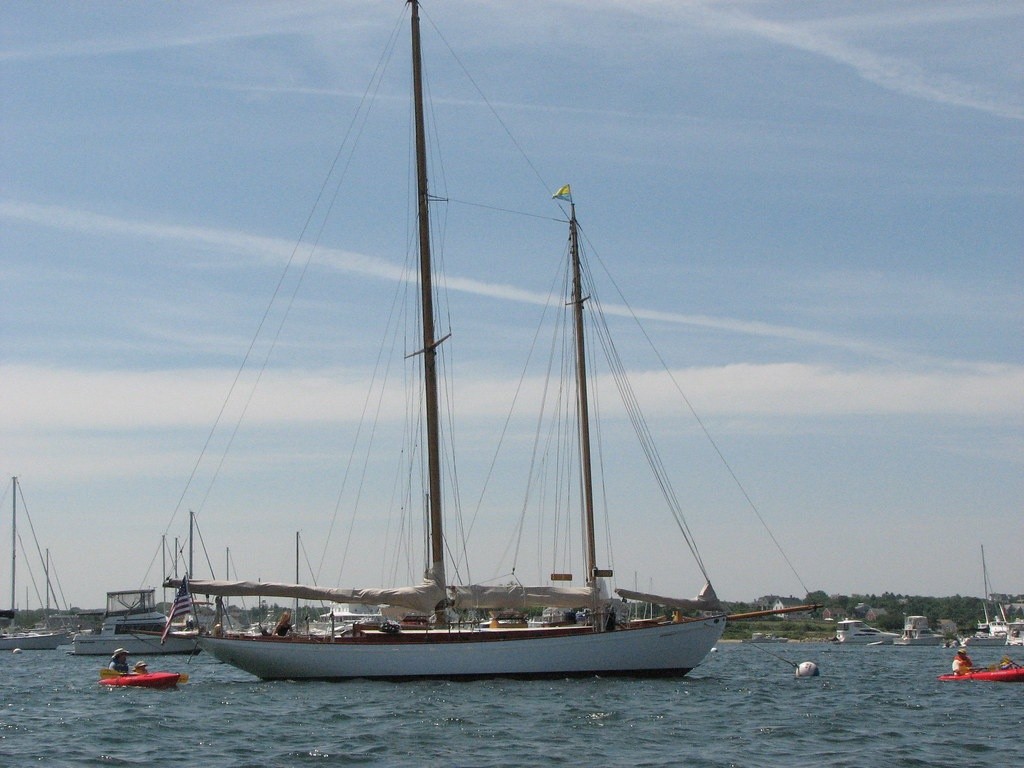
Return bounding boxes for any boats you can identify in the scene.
[1005,621,1024,646]
[834,617,902,645]
[893,616,946,646]
[937,646,1024,683]
[73,587,202,655]
[98,673,178,689]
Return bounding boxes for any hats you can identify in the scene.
[135,661,148,666]
[958,649,966,654]
[112,648,129,658]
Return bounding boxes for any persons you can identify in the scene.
[952,649,972,676]
[108,648,129,672]
[134,662,147,673]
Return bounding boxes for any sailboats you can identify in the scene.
[960,543,1009,646]
[1,478,80,650]
[192,2,823,682]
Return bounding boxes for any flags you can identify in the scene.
[553,185,572,202]
[160,576,191,645]
